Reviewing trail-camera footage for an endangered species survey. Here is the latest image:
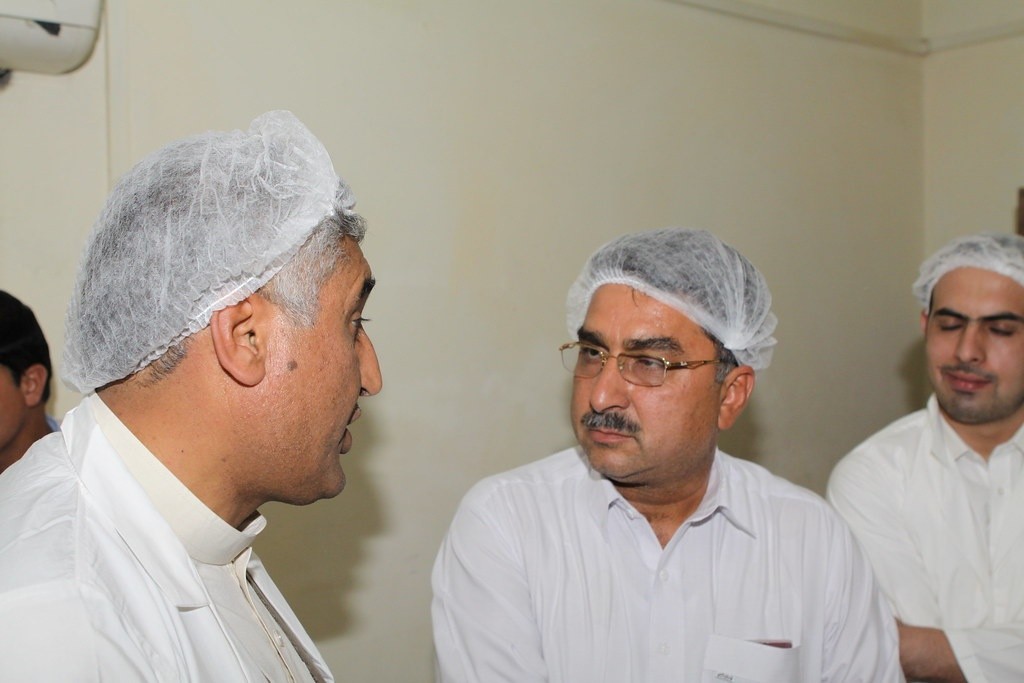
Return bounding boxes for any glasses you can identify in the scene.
[559,341,737,386]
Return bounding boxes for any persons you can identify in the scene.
[0,290,62,476]
[430,228,907,683]
[824,229,1024,683]
[0,110,382,682]
